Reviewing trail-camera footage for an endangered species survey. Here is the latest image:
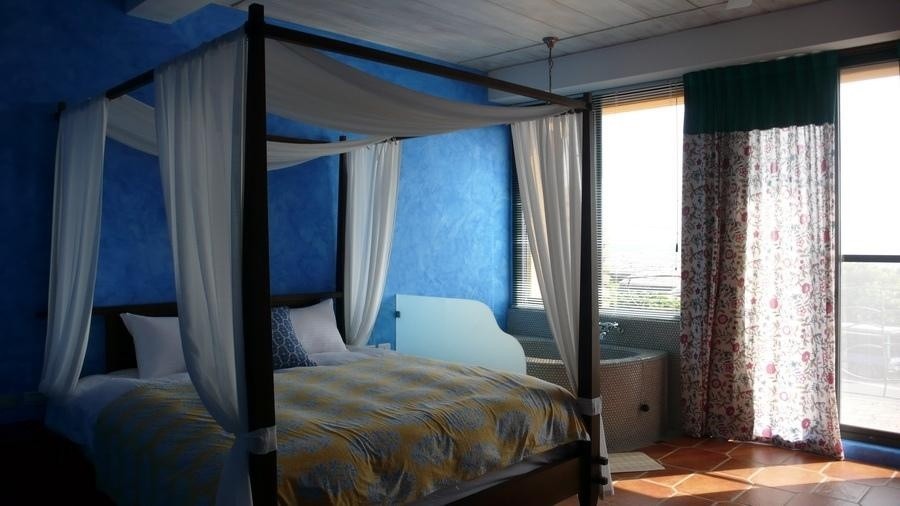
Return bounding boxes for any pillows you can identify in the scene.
[120,296,355,380]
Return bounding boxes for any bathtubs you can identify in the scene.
[509,331,669,456]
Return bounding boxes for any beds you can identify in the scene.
[45,291,602,506]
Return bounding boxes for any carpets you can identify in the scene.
[608,451,666,473]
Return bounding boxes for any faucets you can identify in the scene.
[596,319,626,342]
